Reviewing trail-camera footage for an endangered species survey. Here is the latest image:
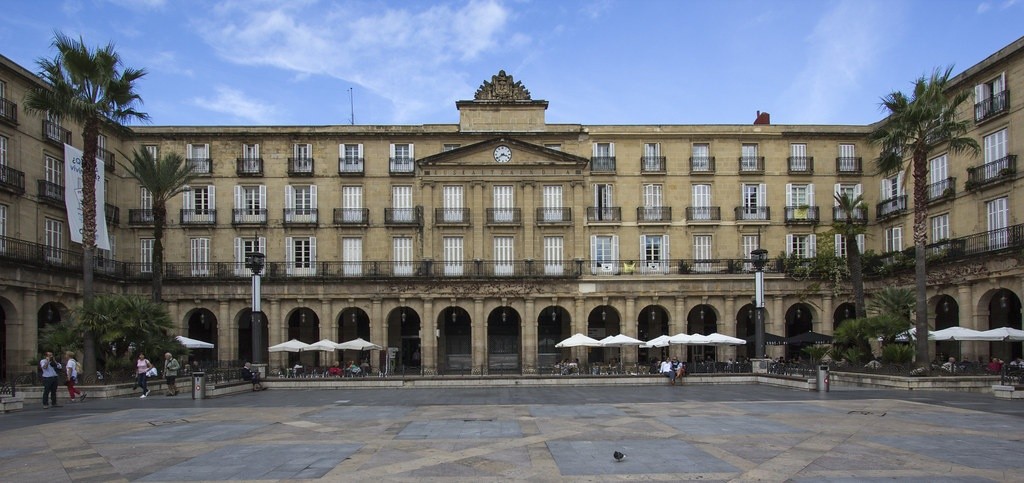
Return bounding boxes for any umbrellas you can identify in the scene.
[555,334,746,374]
[785,331,834,372]
[746,333,789,355]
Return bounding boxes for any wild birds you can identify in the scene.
[614,451,626,461]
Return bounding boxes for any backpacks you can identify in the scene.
[72,360,80,372]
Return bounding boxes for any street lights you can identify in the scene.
[247,252,268,378]
[750,248,771,373]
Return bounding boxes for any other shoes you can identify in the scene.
[262,387,268,390]
[52,404,63,407]
[146,390,150,396]
[175,390,180,396]
[167,393,174,396]
[42,405,48,409]
[80,393,87,401]
[672,381,674,385]
[70,399,79,403]
[140,395,146,399]
[253,389,260,391]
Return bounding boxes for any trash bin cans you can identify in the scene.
[818,365,830,391]
[192,372,206,399]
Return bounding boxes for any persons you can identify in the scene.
[292,361,304,378]
[660,356,684,386]
[135,352,153,399]
[977,357,1004,372]
[164,353,180,396]
[412,348,421,367]
[941,357,956,372]
[1008,354,1024,376]
[959,355,970,370]
[66,351,87,404]
[242,362,269,392]
[330,359,371,378]
[561,361,662,375]
[764,355,809,378]
[682,356,751,377]
[185,357,199,372]
[40,352,62,409]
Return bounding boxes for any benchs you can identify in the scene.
[205,374,819,396]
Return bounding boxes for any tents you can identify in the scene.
[877,327,1024,361]
[175,336,214,372]
[268,339,383,377]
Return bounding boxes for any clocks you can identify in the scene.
[493,145,513,163]
